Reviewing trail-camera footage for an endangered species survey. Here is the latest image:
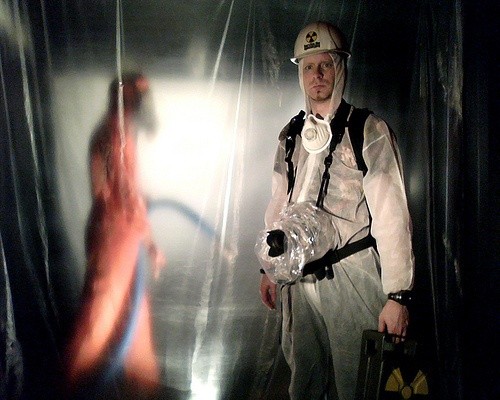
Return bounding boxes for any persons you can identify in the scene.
[259,22,414,400]
[67,73,165,400]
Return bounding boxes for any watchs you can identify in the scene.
[388,290,413,306]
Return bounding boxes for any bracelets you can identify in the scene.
[260,267,266,274]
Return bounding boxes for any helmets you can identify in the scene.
[289,21,352,66]
[109,71,149,112]
